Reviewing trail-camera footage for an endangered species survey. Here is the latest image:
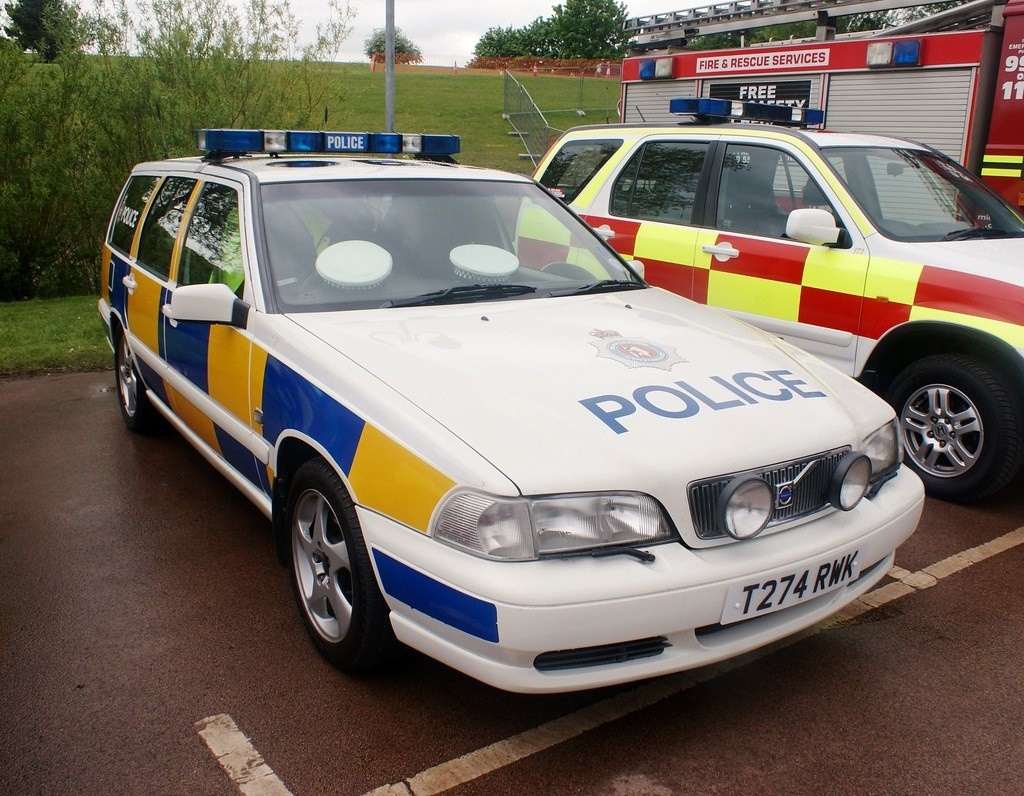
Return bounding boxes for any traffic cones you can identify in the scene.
[533,62,537,76]
[453,61,458,74]
[605,63,611,79]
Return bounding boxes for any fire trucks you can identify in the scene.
[617,0,1024,230]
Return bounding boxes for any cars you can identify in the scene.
[96,127,925,696]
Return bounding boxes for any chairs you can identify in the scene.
[724,171,769,231]
[802,177,829,209]
[263,200,317,285]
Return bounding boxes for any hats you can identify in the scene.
[316,239,394,297]
[449,244,520,287]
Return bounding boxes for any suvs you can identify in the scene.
[513,97,1024,505]
